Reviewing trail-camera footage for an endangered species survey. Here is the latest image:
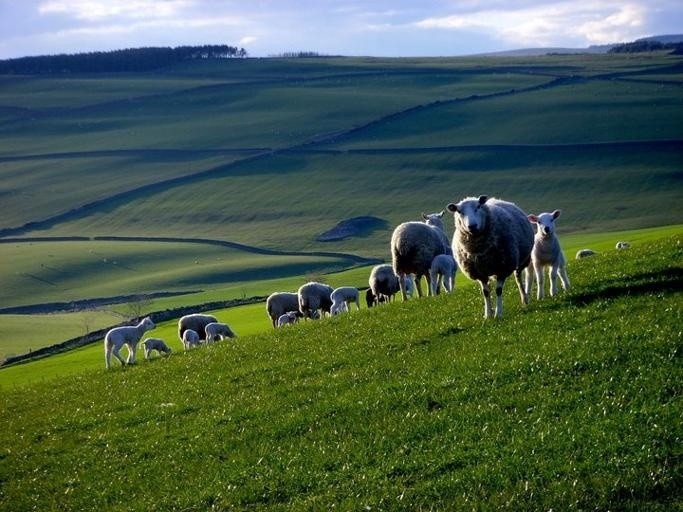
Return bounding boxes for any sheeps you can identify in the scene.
[140,338,171,359]
[265,282,359,329]
[103,316,156,369]
[177,313,236,354]
[523,209,571,301]
[575,249,594,260]
[616,242,630,249]
[365,264,413,308]
[446,195,534,320]
[390,209,449,304]
[430,246,456,297]
